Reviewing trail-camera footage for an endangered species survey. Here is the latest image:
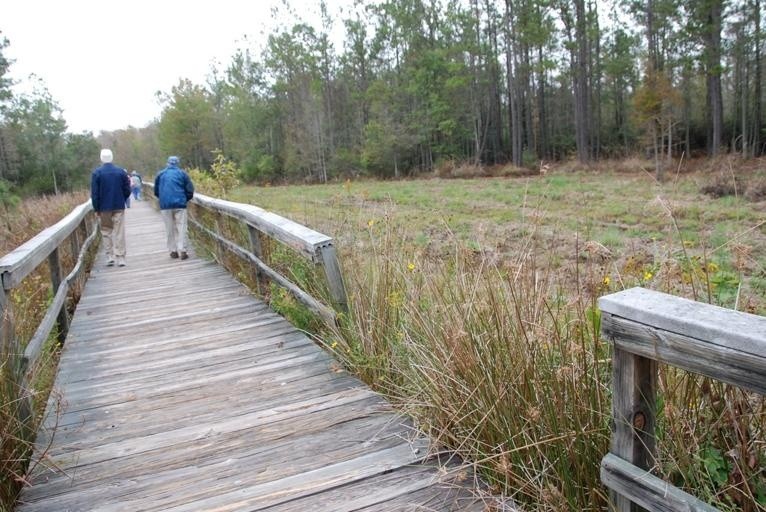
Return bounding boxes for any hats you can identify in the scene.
[167,156,180,165]
[100,148,113,164]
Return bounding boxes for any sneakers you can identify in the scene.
[170,252,189,259]
[106,259,125,266]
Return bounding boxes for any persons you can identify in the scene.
[124,169,142,208]
[154,156,194,260]
[91,149,131,266]
